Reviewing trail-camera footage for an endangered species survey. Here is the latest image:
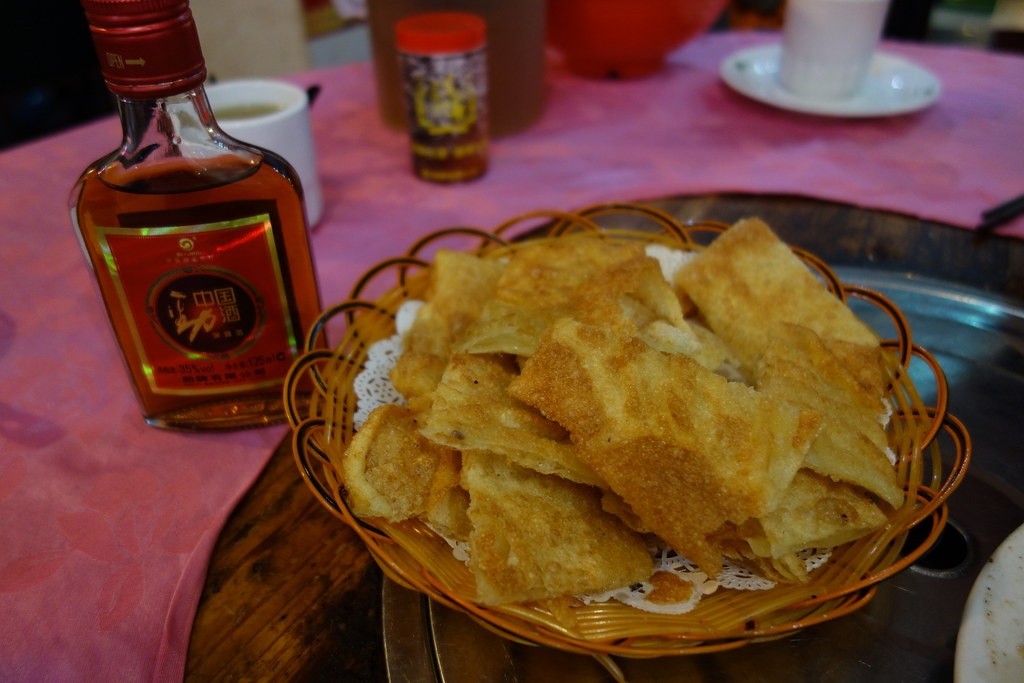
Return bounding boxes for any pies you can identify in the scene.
[350,218,906,603]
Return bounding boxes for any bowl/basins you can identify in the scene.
[546,0,727,80]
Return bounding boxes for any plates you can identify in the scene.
[718,44,942,118]
[953,522,1024,683]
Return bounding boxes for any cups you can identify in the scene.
[169,80,321,228]
[776,0,887,98]
[361,0,549,139]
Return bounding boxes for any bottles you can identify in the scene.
[66,0,327,438]
[392,13,493,184]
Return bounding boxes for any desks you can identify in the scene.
[0,28,1024,683]
